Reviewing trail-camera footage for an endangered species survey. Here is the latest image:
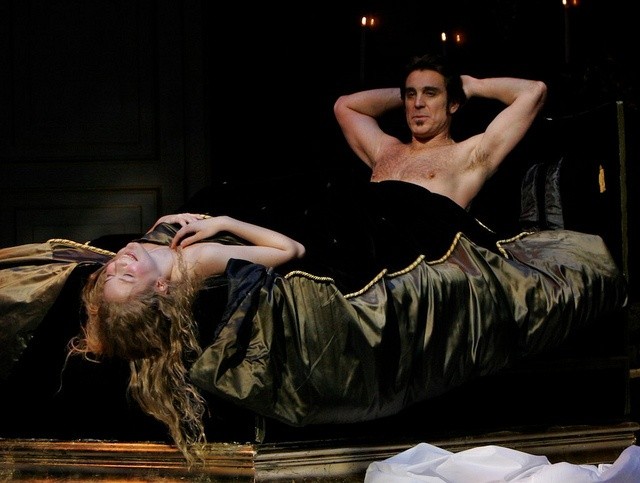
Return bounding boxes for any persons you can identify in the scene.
[333,53,548,208]
[54,211,306,473]
